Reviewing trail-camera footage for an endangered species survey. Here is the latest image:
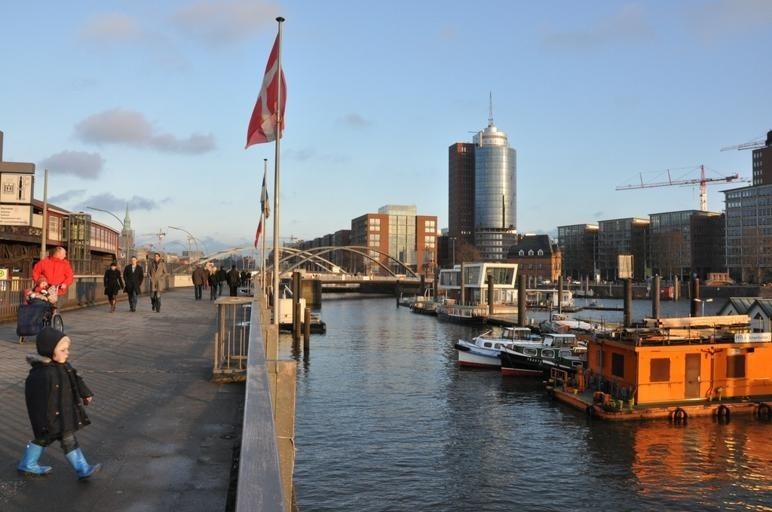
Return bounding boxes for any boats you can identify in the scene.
[272,285,328,335]
[408,289,446,316]
[435,302,490,326]
[452,325,588,369]
[583,299,624,312]
[493,345,588,384]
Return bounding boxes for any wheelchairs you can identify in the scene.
[16,284,65,344]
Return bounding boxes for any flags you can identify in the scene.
[244,31,287,150]
[254,219,263,249]
[259,173,270,218]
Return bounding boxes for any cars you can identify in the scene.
[540,278,551,284]
[572,280,581,284]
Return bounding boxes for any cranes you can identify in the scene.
[612,162,741,213]
[141,228,167,245]
[718,128,771,154]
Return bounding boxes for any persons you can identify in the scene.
[29,272,50,322]
[32,246,73,330]
[103,263,124,311]
[192,264,251,301]
[16,328,104,482]
[146,253,168,313]
[123,255,144,312]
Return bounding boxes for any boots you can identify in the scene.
[64,445,102,480]
[17,440,53,476]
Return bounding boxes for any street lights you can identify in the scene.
[86,204,129,267]
[449,236,457,266]
[694,298,714,316]
[167,224,200,261]
